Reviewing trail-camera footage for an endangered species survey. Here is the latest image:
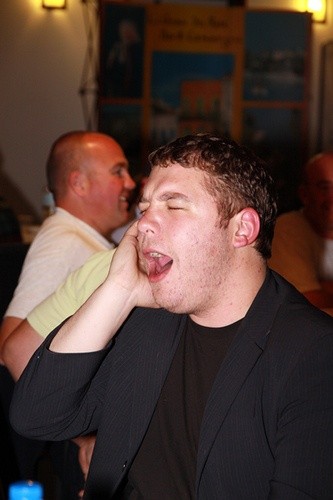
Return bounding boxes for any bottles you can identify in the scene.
[42,184,56,218]
[8,479,43,500]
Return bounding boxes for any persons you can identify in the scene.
[265,151,333,311]
[0,131,135,364]
[7,132,333,500]
[2,243,135,481]
